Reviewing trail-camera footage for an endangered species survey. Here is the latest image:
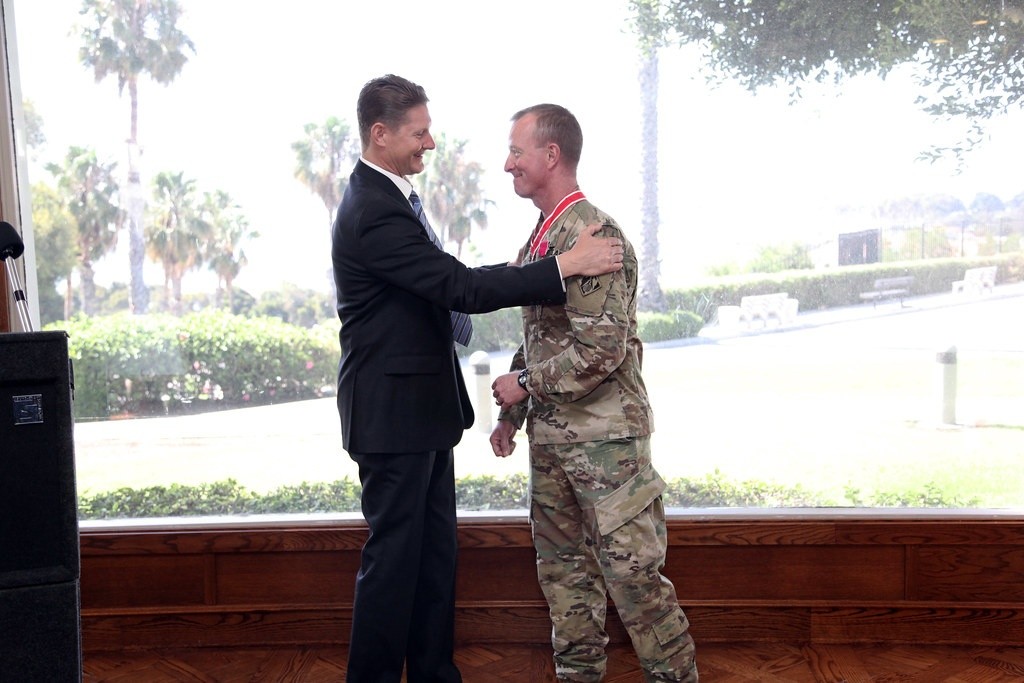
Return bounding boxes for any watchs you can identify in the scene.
[518,369,528,393]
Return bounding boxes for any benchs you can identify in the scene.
[739,290,789,330]
[951,266,997,296]
[859,276,916,311]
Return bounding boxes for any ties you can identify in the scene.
[408,190,474,348]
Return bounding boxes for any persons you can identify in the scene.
[331,74,624,683]
[489,105,699,683]
